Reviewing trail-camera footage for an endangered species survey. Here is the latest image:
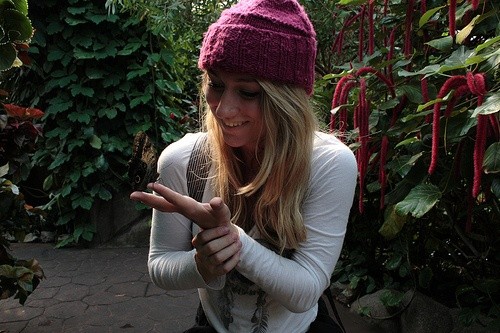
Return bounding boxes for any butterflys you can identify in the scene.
[128,128,173,200]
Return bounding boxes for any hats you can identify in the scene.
[198,0,316,95]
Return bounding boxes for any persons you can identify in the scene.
[130,0,359,333]
